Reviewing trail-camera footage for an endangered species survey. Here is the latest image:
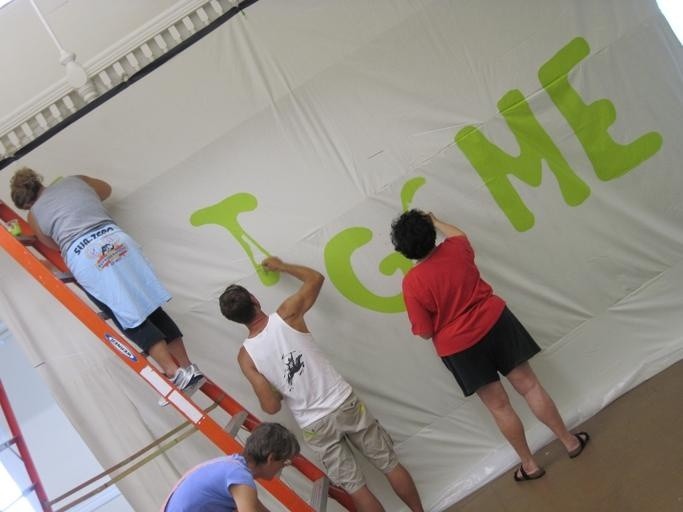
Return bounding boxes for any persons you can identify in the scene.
[160,421,300,512]
[389,208,590,480]
[10,166,207,407]
[218,255,425,512]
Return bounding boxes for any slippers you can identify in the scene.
[514,465,545,481]
[570,432,588,458]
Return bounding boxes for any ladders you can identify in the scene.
[0,198,358,512]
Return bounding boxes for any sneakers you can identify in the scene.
[159,364,207,407]
